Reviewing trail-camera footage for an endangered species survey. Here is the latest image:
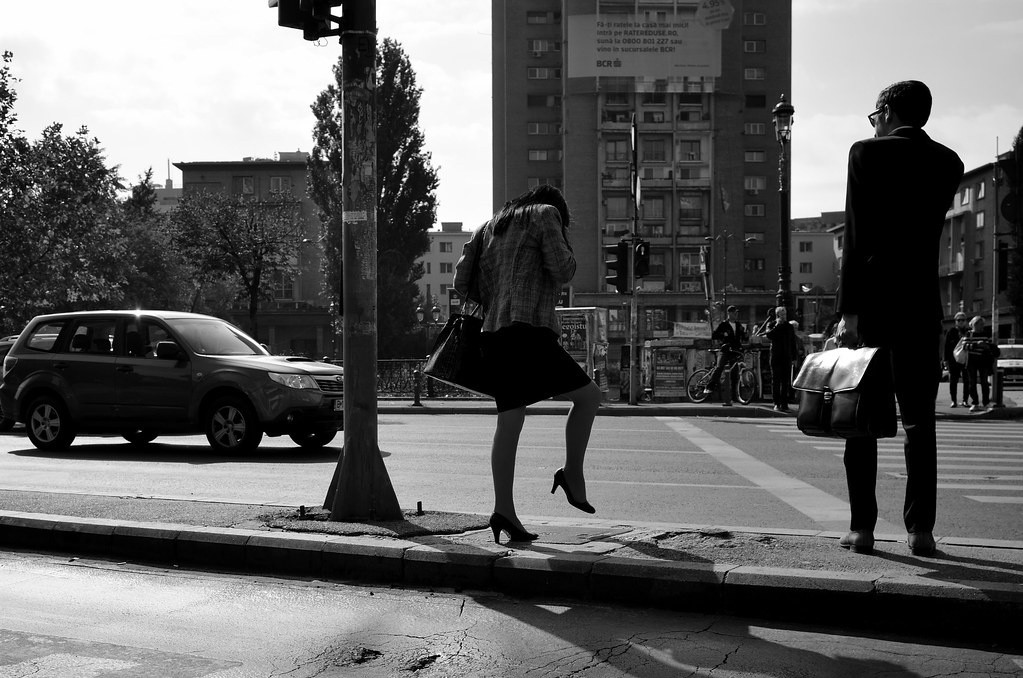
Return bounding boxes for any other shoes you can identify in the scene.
[703,389,713,393]
[984,404,990,411]
[951,403,957,407]
[970,405,978,411]
[781,407,792,413]
[962,401,968,406]
[774,404,780,411]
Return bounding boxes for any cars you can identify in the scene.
[988,339,1023,387]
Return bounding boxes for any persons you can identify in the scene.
[765,306,799,413]
[571,330,581,341]
[943,312,972,408]
[703,305,749,402]
[788,320,804,403]
[453,185,602,543]
[824,322,840,350]
[833,80,964,555]
[963,316,996,412]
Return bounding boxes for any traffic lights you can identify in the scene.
[603,241,628,294]
[268,0,331,41]
[997,238,1013,294]
[632,240,651,277]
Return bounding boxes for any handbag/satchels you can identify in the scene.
[423,314,495,400]
[953,338,967,364]
[791,334,897,438]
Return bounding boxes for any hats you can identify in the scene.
[727,306,739,313]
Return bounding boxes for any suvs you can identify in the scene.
[0,310,345,459]
[0,332,157,433]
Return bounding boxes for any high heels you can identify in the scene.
[551,467,596,513]
[489,511,538,543]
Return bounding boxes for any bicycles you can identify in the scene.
[686,348,758,406]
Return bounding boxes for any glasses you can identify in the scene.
[956,319,965,322]
[868,108,884,127]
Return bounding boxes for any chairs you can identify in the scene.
[95,339,111,350]
[127,330,145,355]
[72,333,89,351]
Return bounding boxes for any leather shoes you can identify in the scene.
[839,530,875,553]
[907,532,936,555]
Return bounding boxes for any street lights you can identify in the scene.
[415,281,441,398]
[704,229,758,321]
[772,93,797,402]
[301,237,337,357]
